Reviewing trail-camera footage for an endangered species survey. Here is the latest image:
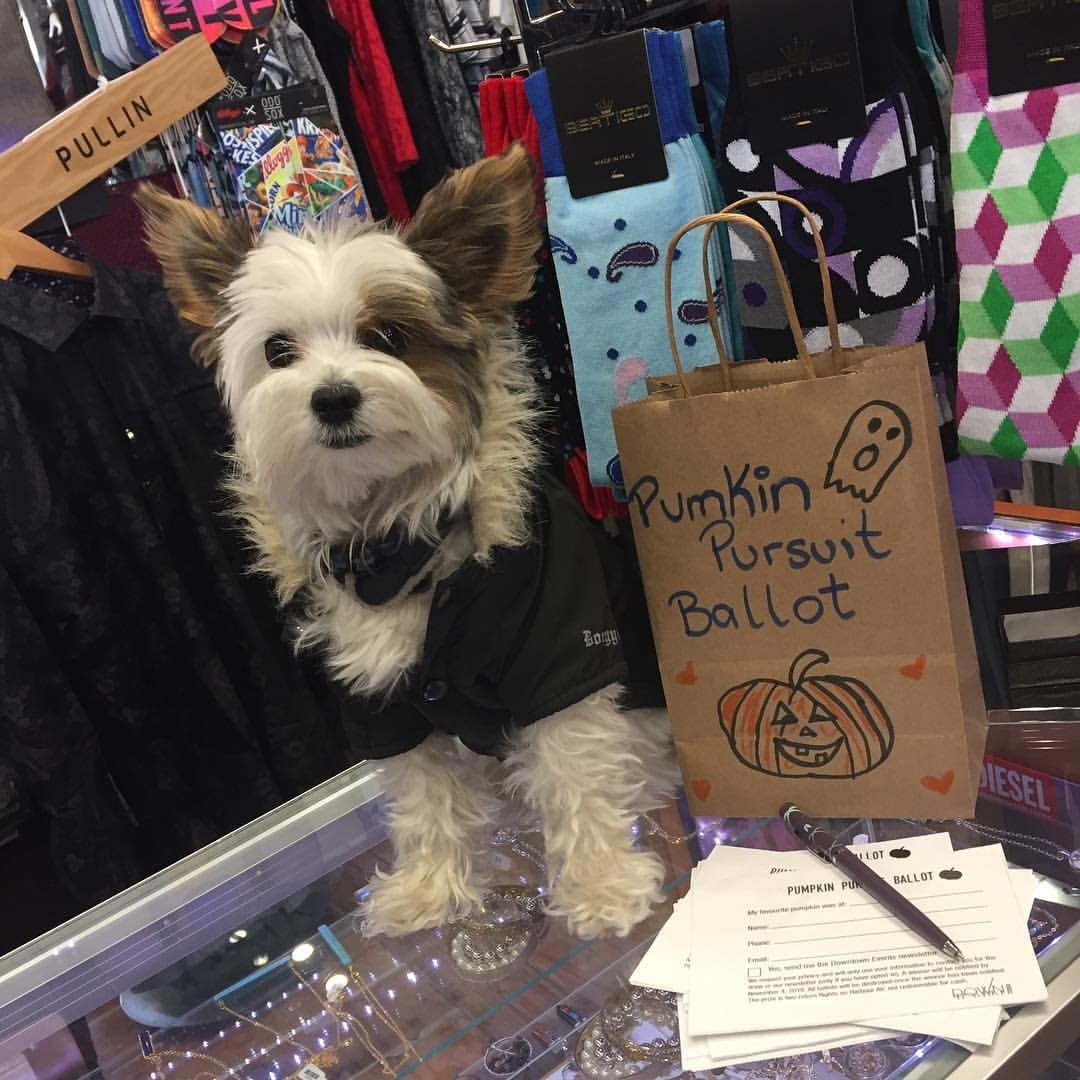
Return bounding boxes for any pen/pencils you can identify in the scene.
[779,802,965,963]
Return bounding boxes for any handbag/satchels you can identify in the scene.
[606,190,990,822]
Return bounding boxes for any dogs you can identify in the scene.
[134,144,679,937]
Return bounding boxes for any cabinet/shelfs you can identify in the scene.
[1,750,1080,1080]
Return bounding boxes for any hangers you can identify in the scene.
[0,230,89,281]
